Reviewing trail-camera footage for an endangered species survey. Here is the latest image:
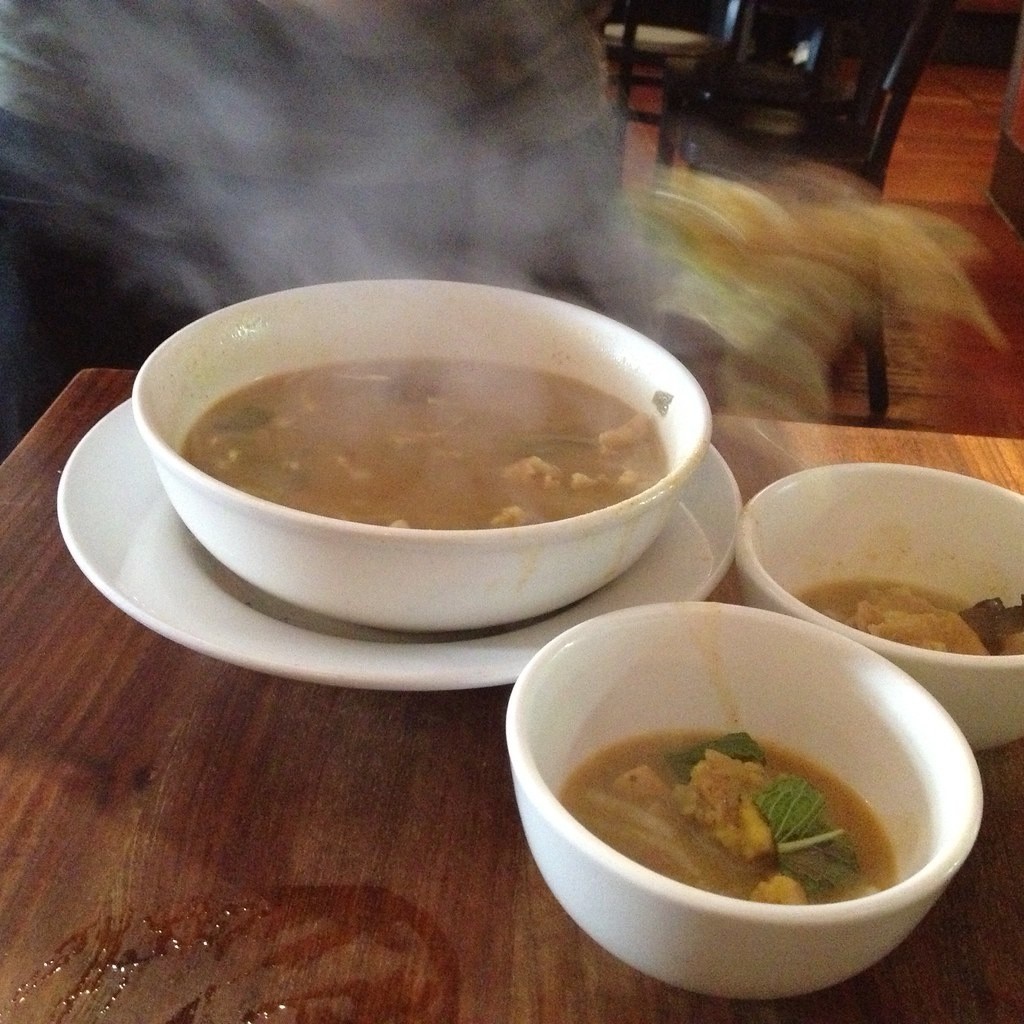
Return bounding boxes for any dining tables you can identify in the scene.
[0,369,1022,1024]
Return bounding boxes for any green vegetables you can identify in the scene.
[665,731,863,898]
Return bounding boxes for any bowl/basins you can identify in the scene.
[507,602,984,997]
[739,460,1024,758]
[132,279,715,633]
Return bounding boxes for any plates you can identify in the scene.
[56,388,745,694]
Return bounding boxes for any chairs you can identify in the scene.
[603,0,952,413]
